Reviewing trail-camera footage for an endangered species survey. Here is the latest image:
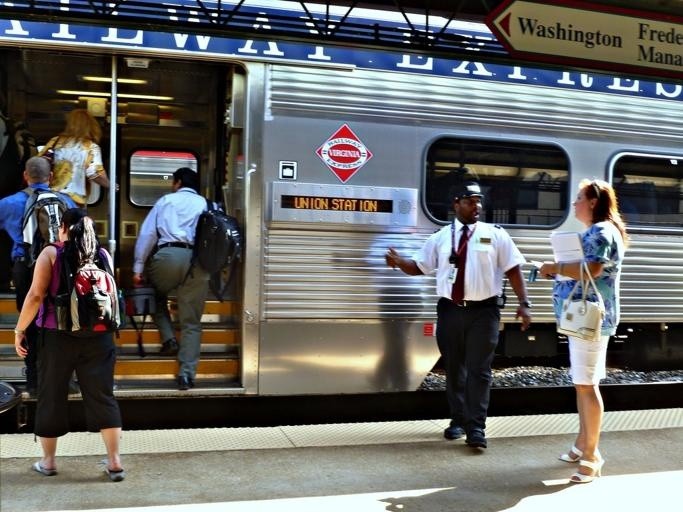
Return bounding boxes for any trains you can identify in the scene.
[0,32,681,428]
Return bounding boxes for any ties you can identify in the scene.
[451,225,468,305]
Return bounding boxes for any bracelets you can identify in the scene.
[13,330,24,334]
[559,261,564,274]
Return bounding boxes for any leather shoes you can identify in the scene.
[443,424,466,438]
[176,375,192,390]
[465,426,488,449]
[161,337,180,356]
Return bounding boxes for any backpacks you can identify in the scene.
[193,199,242,271]
[18,187,70,268]
[0,114,39,185]
[43,240,125,338]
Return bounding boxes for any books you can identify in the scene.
[551,229,585,282]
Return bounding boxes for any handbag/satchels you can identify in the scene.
[41,147,54,168]
[557,261,605,343]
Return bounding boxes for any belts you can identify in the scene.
[14,256,25,263]
[158,242,193,249]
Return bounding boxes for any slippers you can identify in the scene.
[104,464,126,482]
[31,461,58,475]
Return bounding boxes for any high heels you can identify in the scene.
[570,457,605,483]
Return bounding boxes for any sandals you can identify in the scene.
[557,445,584,463]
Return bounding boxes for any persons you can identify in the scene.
[1,156,80,400]
[131,168,224,391]
[539,178,628,482]
[39,107,120,218]
[386,182,533,448]
[0,113,23,293]
[13,208,126,482]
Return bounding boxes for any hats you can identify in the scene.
[449,181,484,199]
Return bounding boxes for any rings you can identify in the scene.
[541,268,544,271]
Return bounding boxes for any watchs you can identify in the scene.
[519,300,533,308]
